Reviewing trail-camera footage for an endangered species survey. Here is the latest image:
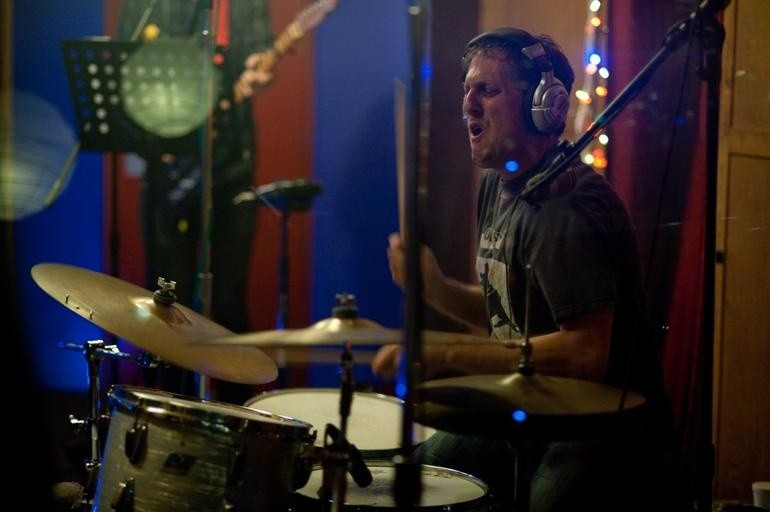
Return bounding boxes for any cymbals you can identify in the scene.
[208,313,473,348]
[30,259,281,387]
[410,368,649,421]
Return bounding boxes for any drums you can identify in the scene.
[87,386,314,512]
[288,457,496,512]
[240,386,444,466]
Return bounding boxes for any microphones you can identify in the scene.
[325,423,372,487]
[236,179,323,216]
[525,167,576,201]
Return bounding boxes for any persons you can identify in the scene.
[372,35,675,512]
[113,0,276,407]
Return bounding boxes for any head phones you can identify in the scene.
[463,26,569,137]
